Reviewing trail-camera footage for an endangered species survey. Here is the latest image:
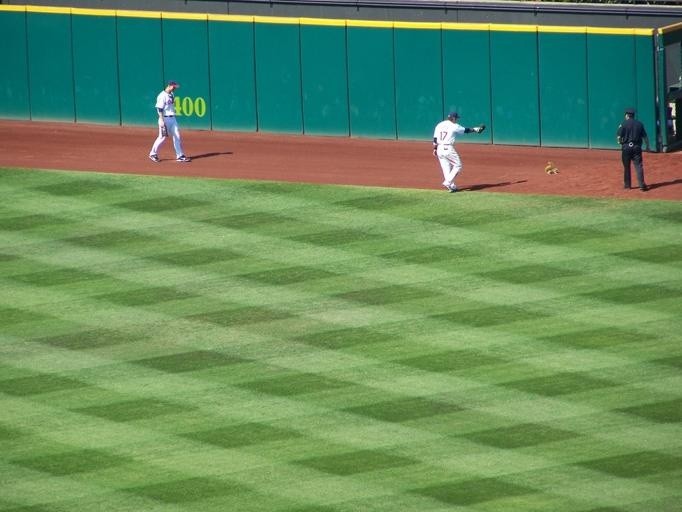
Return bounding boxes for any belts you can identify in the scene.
[162,115,175,118]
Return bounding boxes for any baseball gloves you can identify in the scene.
[545,160,559,176]
[161,125,168,138]
[477,125,485,133]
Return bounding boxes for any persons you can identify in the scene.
[613,107,653,192]
[147,78,193,164]
[430,112,487,192]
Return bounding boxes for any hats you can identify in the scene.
[625,108,636,114]
[168,81,179,88]
[447,112,460,119]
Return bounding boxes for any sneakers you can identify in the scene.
[176,155,191,162]
[148,154,161,163]
[442,181,457,192]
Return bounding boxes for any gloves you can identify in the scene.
[473,126,483,134]
[432,149,439,158]
